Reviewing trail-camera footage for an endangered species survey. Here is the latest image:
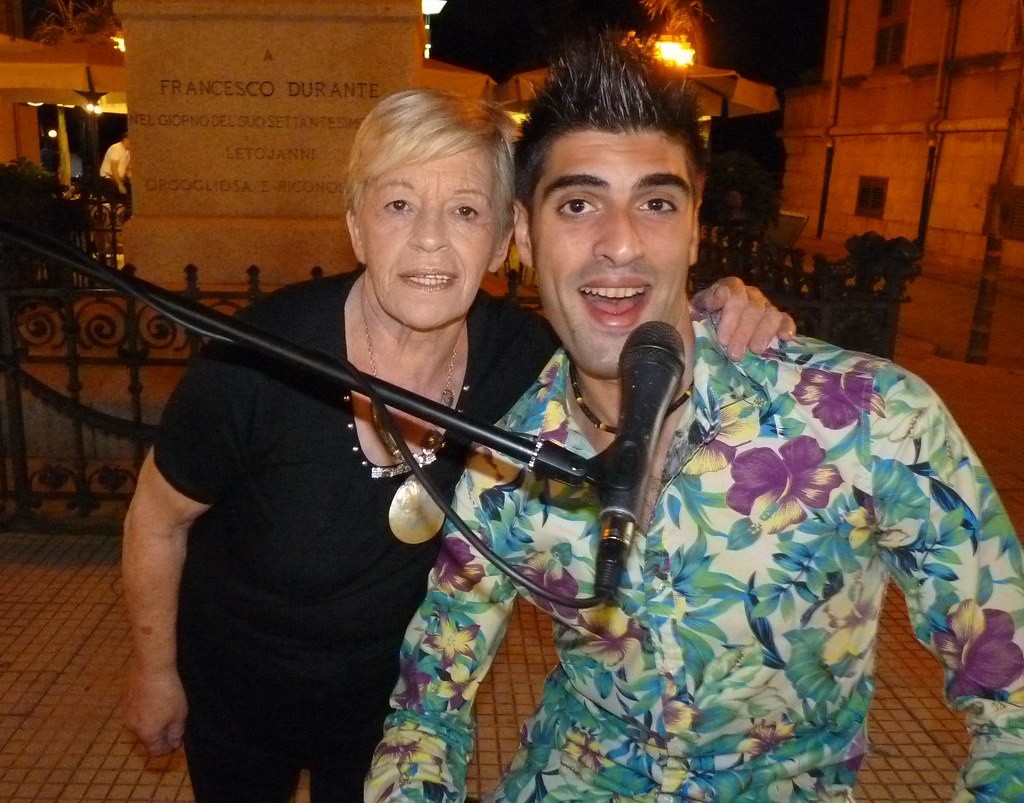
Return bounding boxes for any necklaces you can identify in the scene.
[358,279,461,460]
[566,359,696,435]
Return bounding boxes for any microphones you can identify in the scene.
[595,322,687,599]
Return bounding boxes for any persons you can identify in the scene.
[98,132,131,225]
[362,41,1023,803]
[123,91,797,803]
